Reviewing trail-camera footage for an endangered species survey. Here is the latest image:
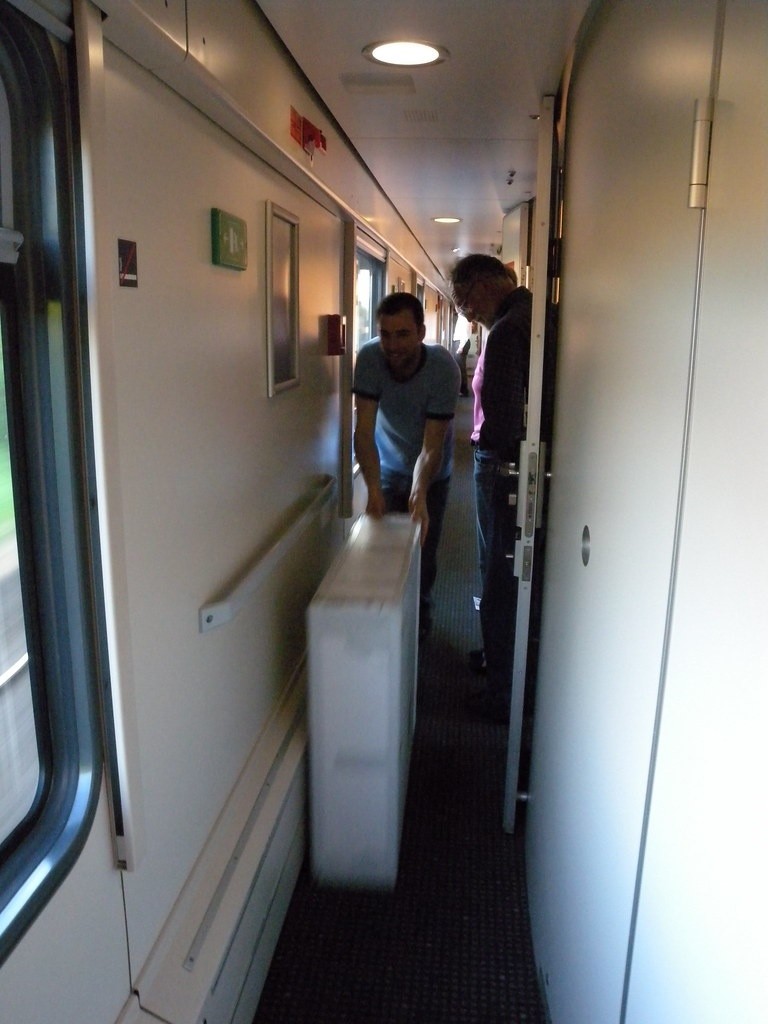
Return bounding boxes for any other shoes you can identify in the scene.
[462,648,499,674]
[419,629,427,644]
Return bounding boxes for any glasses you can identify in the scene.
[454,281,475,316]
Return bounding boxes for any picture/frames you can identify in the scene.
[265,200,300,398]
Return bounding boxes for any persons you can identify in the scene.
[353,292,462,644]
[448,253,560,725]
